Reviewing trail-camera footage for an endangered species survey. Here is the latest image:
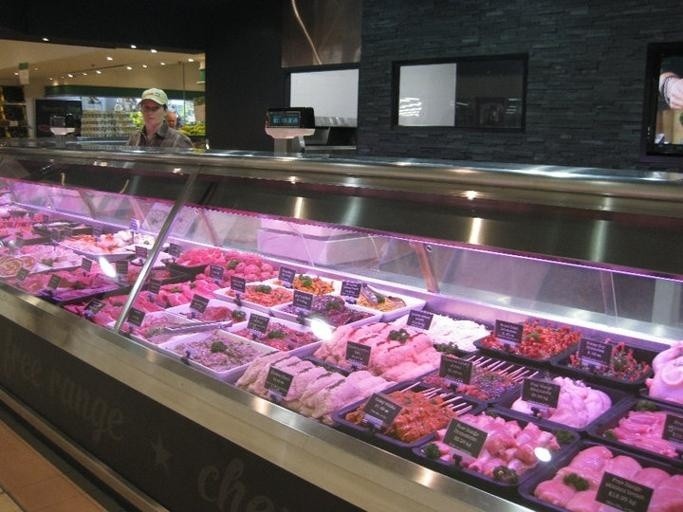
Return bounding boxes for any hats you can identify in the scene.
[137,88,168,105]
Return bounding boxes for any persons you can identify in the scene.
[164,112,177,129]
[125,88,194,147]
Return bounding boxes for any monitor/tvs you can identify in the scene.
[638,40,683,174]
[35,99,82,137]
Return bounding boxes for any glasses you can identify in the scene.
[139,105,160,113]
